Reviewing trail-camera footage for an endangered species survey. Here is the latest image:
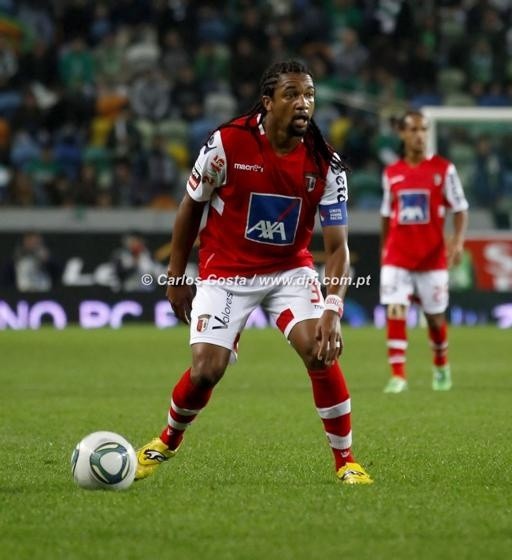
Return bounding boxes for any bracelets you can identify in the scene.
[323,293,345,318]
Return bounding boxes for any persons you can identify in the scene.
[378,111,471,390]
[0,0,512,293]
[132,58,375,488]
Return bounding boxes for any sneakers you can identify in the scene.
[336,462,374,486]
[384,375,408,395]
[432,363,456,392]
[133,438,186,481]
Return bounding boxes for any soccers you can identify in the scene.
[71,430,138,491]
